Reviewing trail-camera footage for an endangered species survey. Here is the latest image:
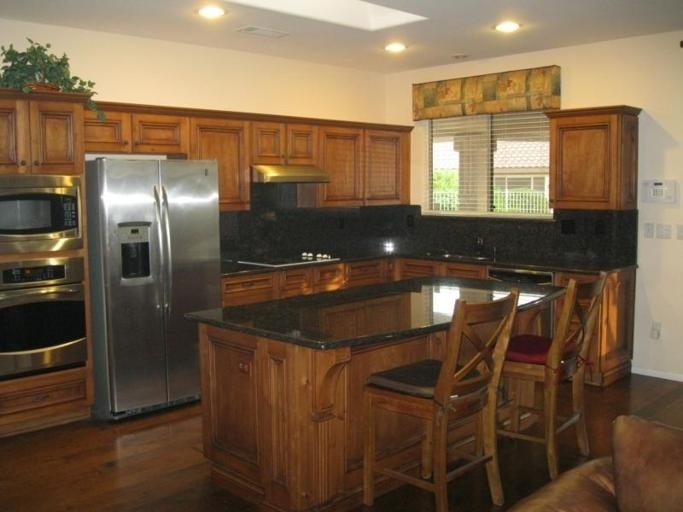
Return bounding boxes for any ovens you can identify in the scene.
[0,174,87,384]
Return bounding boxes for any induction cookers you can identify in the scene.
[237,253,340,269]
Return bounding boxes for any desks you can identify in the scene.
[182,275,576,512]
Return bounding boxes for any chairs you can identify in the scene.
[476,266,613,483]
[509,413,683,511]
[356,282,524,511]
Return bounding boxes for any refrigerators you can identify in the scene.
[87,157,221,424]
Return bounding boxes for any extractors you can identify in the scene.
[248,163,330,184]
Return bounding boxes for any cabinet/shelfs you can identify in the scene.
[249,116,317,163]
[221,271,278,308]
[278,264,346,300]
[395,256,486,284]
[490,265,635,389]
[0,93,84,174]
[319,123,413,205]
[83,103,191,155]
[346,259,395,294]
[549,112,640,208]
[193,114,251,212]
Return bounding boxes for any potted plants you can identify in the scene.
[0,34,105,127]
